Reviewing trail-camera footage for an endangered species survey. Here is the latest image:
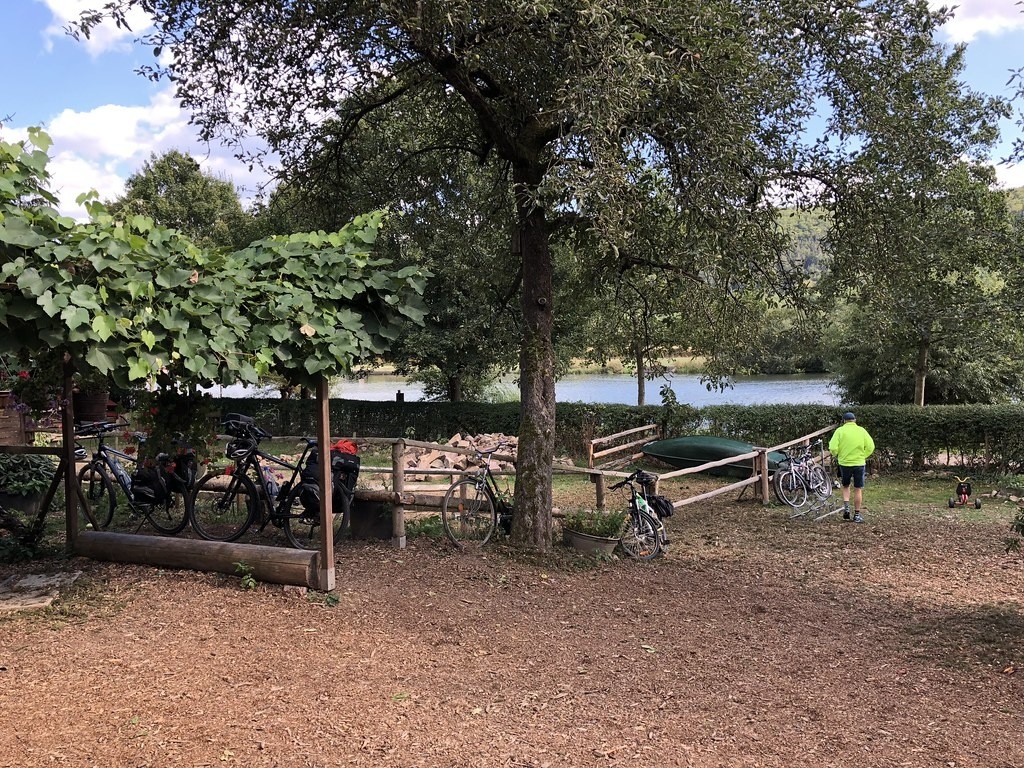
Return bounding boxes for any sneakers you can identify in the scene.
[843,512,850,521]
[853,515,864,522]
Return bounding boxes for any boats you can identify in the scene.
[642,435,801,479]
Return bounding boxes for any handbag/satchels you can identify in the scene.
[638,492,674,518]
[300,440,361,514]
[130,453,196,504]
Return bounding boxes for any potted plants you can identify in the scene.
[0,444,56,515]
[558,506,624,554]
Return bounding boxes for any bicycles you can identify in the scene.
[189,420,355,552]
[442,439,517,549]
[608,470,671,561]
[772,436,832,508]
[73,415,190,534]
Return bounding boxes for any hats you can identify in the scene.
[843,413,855,419]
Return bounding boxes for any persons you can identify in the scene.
[829,412,875,522]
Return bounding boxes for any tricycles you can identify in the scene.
[949,475,982,510]
